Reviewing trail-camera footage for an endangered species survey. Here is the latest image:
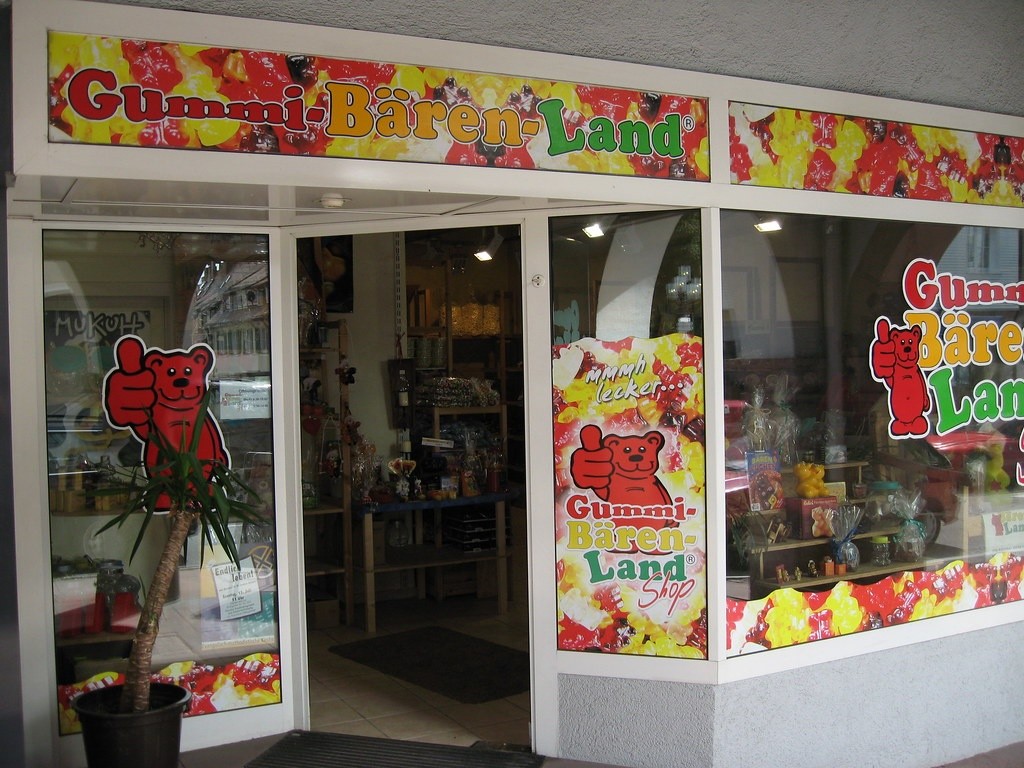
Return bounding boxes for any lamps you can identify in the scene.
[582,214,617,238]
[480,303,500,335]
[460,303,483,335]
[473,226,505,262]
[441,303,463,335]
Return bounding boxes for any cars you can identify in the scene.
[725,356,1024,554]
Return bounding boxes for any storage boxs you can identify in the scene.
[446,511,512,554]
[356,518,386,565]
[305,583,339,630]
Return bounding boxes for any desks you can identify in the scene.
[324,488,520,632]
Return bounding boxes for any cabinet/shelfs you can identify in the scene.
[725,409,986,599]
[402,306,511,499]
[298,317,354,627]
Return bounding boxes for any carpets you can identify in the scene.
[328,625,530,705]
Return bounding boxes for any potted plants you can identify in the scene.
[68,380,271,768]
